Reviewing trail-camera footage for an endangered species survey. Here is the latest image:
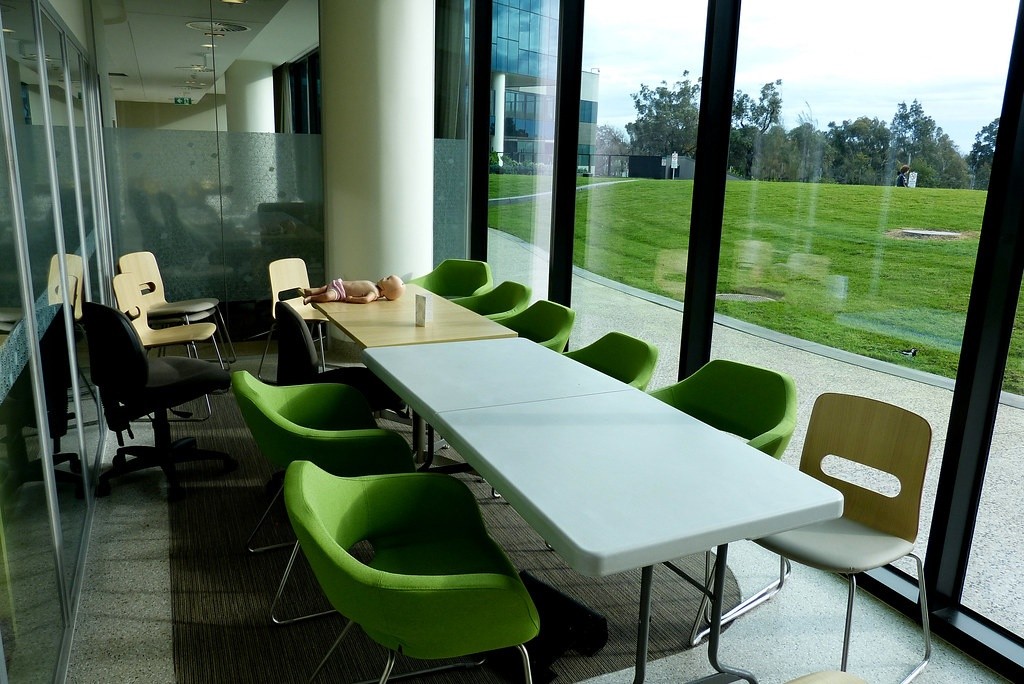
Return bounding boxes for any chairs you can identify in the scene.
[113,272,227,422]
[563,330,659,394]
[231,368,417,558]
[0,303,84,503]
[450,279,532,321]
[82,301,231,502]
[267,458,541,684]
[496,298,577,354]
[130,170,324,294]
[274,300,411,417]
[408,259,494,300]
[686,389,934,684]
[115,262,231,376]
[654,235,847,323]
[46,252,86,321]
[119,250,238,364]
[647,359,799,460]
[258,257,331,382]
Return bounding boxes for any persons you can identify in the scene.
[302,273,406,306]
[896,166,910,188]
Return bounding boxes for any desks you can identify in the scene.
[362,335,847,684]
[298,283,519,351]
